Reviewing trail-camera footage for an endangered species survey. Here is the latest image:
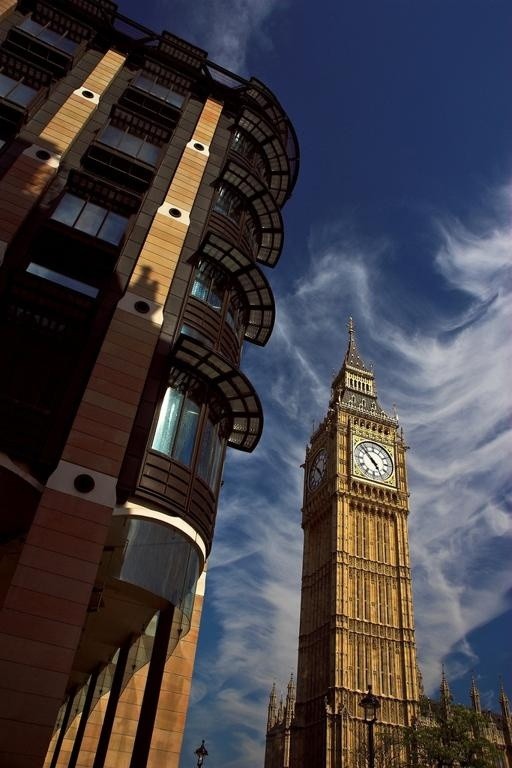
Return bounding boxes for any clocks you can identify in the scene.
[307,447,329,493]
[354,439,395,482]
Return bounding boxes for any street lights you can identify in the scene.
[360,685,382,767]
[194,738,208,766]
